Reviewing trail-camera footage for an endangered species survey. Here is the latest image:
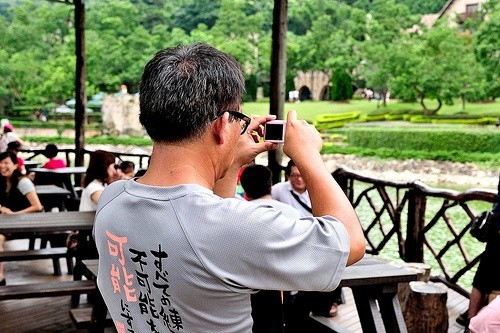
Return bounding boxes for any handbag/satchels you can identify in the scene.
[470,211,494,239]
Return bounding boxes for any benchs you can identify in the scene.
[0,185,114,333]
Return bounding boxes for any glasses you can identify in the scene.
[218,110,251,135]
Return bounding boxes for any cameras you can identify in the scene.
[264,120,286,143]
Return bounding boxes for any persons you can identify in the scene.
[29,144,66,181]
[92,42,366,333]
[0,124,23,153]
[76,150,135,251]
[456,172,500,333]
[0,151,42,286]
[29,106,47,122]
[238,159,346,318]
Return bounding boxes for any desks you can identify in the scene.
[23,161,42,175]
[0,211,96,308]
[331,263,419,333]
[28,167,87,199]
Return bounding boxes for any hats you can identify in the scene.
[4,124,13,132]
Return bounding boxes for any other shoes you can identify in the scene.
[0,279,6,286]
[330,305,337,317]
[456,310,470,327]
